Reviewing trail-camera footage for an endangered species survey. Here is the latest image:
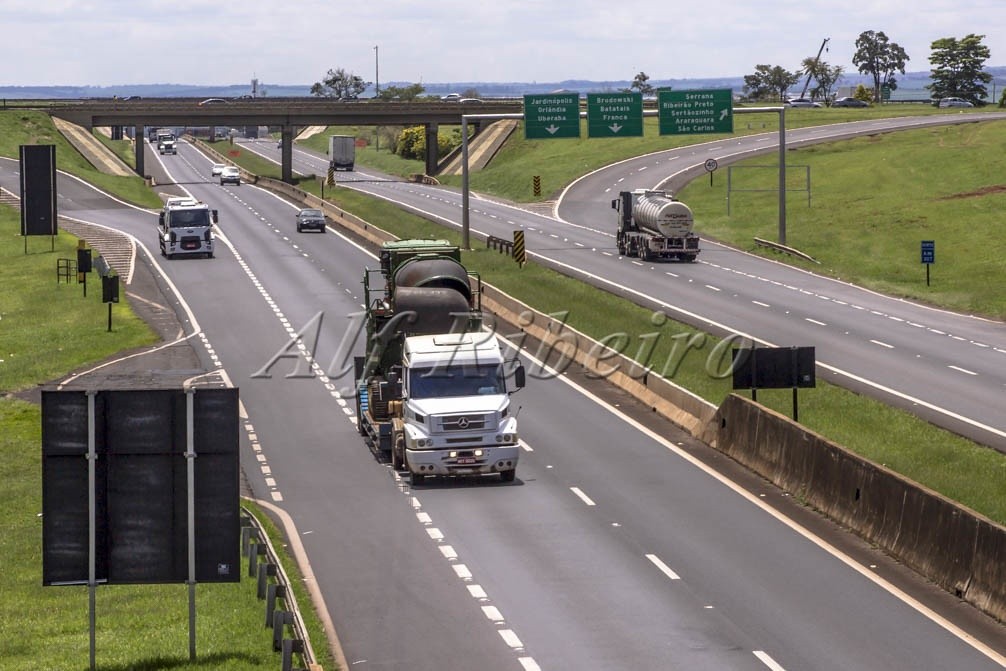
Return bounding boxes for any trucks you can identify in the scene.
[147,127,177,155]
[326,134,356,171]
[156,191,219,259]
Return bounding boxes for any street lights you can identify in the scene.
[373,45,379,99]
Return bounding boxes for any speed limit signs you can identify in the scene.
[704,159,718,171]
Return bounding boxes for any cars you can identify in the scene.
[124,95,142,101]
[220,166,241,186]
[938,97,974,108]
[830,96,870,108]
[782,98,823,109]
[459,98,485,104]
[338,94,359,104]
[440,93,463,102]
[295,208,329,233]
[198,98,229,107]
[232,94,253,100]
[211,163,226,177]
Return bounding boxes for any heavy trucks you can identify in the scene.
[611,188,701,262]
[351,236,526,488]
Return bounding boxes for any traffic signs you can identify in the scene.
[657,88,734,136]
[586,92,644,138]
[523,92,581,139]
